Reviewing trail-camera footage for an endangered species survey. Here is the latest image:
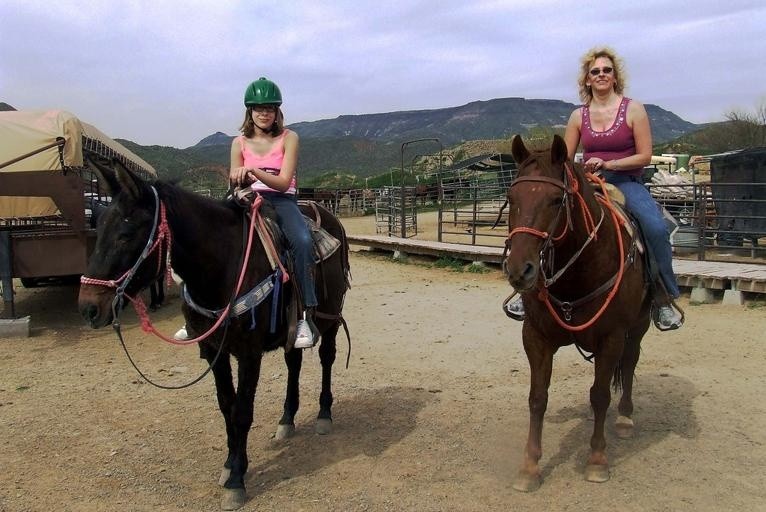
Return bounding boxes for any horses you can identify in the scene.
[413,178,471,207]
[315,188,377,215]
[502,132,656,493]
[77,155,349,511]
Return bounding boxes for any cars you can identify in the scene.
[652,175,713,225]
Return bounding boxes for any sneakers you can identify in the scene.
[174,323,192,340]
[659,303,682,326]
[294,319,315,349]
[504,296,525,316]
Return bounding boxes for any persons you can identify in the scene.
[504,46,682,329]
[173,77,317,350]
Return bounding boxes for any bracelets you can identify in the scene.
[612,160,618,171]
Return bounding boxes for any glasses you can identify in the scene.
[588,68,612,75]
[249,103,278,112]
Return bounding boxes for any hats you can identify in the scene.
[243,76,283,105]
